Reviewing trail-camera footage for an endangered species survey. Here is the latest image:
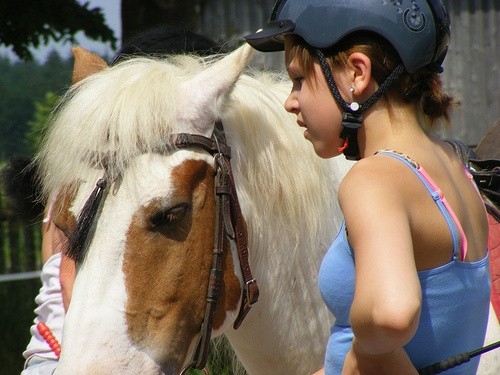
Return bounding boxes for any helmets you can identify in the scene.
[244,0,451,74]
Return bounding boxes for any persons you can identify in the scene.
[244,0,490,375]
[21,26,220,375]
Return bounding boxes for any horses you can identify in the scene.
[33,28,361,375]
